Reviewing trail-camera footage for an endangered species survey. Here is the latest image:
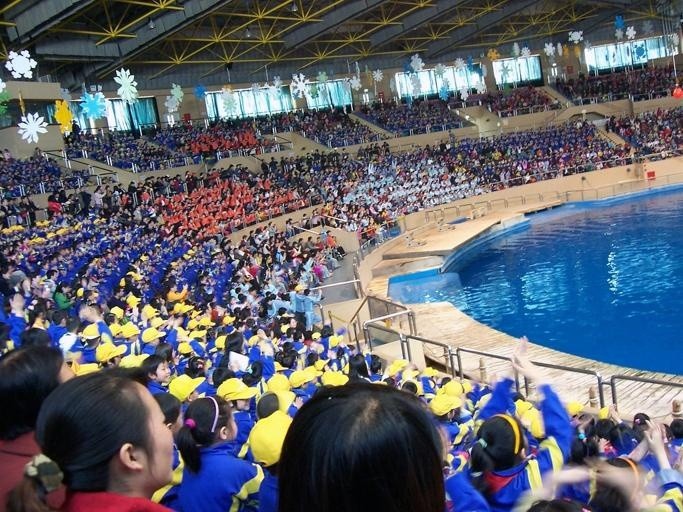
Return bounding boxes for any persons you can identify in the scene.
[277,332,682,512]
[0,102,340,512]
[338,51,682,246]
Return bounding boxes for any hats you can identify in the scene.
[36,220,52,228]
[62,298,350,411]
[388,358,463,416]
[2,224,26,235]
[249,411,294,468]
[27,236,47,246]
[47,228,70,238]
